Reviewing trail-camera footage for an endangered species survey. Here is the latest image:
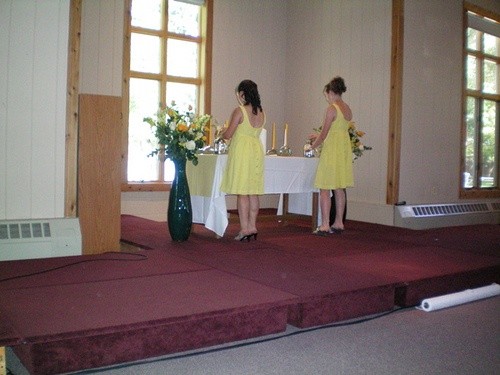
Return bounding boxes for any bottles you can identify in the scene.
[311,138,319,158]
[214,137,220,154]
[266,148,278,155]
[278,146,289,156]
[219,140,225,154]
[304,140,312,157]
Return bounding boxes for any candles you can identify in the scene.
[284,124,289,144]
[273,123,276,150]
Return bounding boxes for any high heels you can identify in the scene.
[246,233,258,240]
[234,231,251,242]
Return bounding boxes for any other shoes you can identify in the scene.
[312,227,330,235]
[331,226,345,233]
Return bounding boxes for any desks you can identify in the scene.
[186,153,333,240]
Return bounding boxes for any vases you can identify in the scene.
[329,189,348,224]
[167,158,193,244]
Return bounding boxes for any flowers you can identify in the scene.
[305,120,372,164]
[142,100,213,165]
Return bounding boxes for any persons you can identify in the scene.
[221,79,266,242]
[308,77,353,235]
